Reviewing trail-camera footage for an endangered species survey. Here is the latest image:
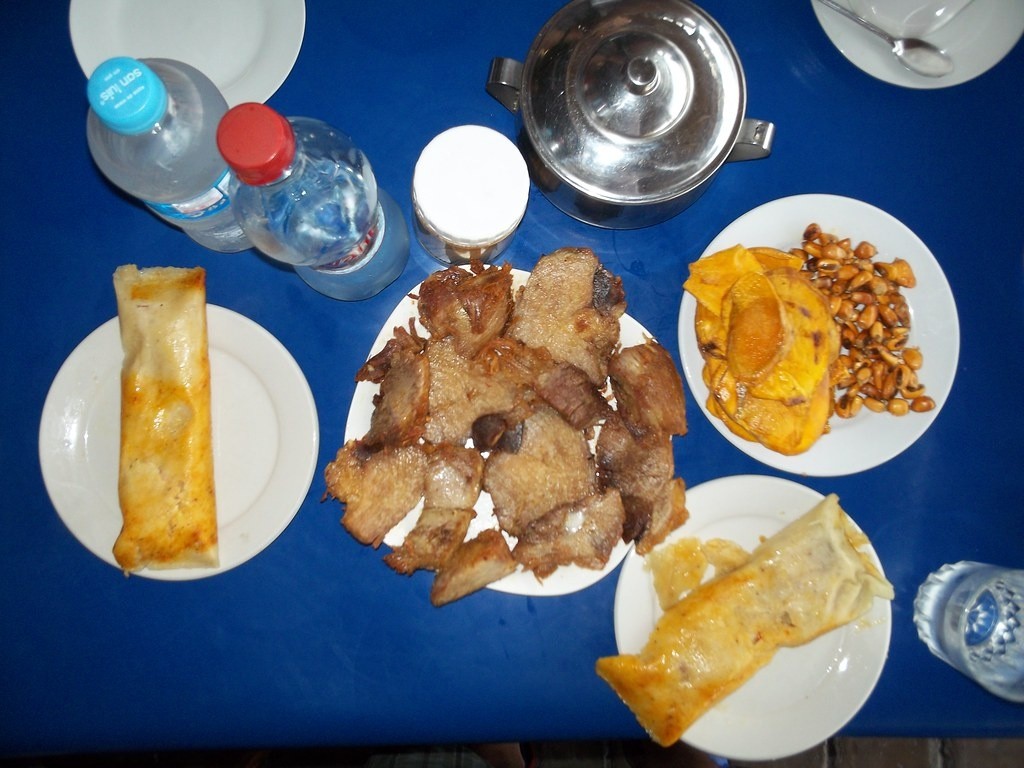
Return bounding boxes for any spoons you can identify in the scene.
[816,0,956,79]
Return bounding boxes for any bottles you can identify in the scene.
[414,123,530,266]
[84,57,271,255]
[216,104,410,299]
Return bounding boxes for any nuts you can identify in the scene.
[790,223,935,419]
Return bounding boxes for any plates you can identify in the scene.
[39,302,319,580]
[810,0,1024,92]
[611,469,893,761]
[66,0,306,130]
[344,262,675,597]
[677,192,961,475]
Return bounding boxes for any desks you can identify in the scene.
[0,0,1024,755]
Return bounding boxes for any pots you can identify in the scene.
[486,0,775,230]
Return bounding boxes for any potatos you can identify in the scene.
[683,245,840,454]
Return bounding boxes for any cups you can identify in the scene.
[914,556,1024,703]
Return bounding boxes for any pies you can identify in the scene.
[112,263,220,575]
[595,494,893,747]
